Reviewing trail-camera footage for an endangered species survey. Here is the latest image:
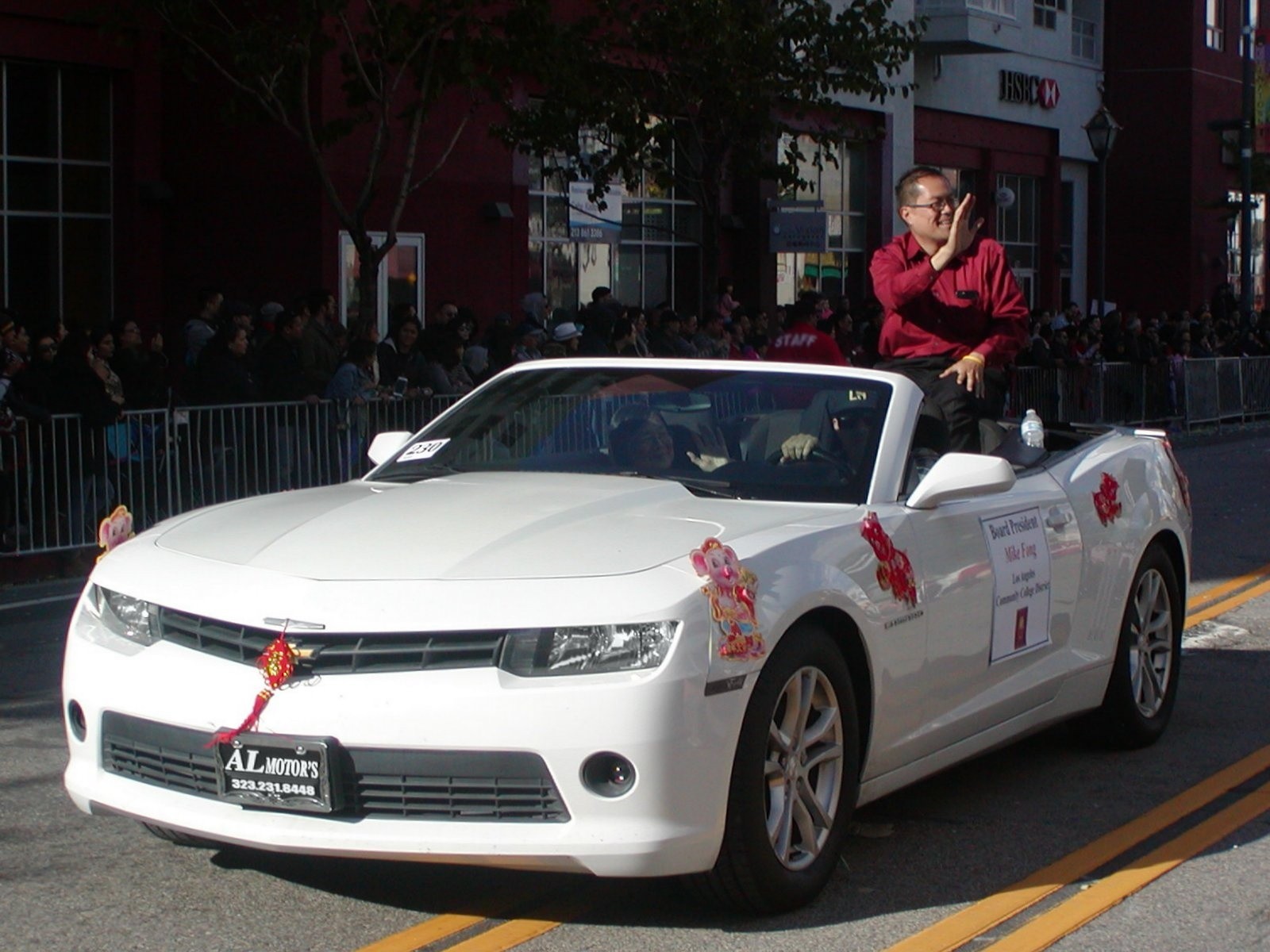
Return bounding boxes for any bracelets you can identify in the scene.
[963,355,985,368]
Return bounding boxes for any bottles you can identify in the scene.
[1021,409,1044,448]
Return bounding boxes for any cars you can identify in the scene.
[58,357,1194,920]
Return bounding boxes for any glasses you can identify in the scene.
[543,302,549,309]
[460,325,471,331]
[906,198,960,212]
[38,342,58,353]
[122,327,140,334]
[837,413,880,430]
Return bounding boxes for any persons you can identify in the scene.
[171,289,389,510]
[0,311,178,574]
[646,273,884,418]
[386,287,656,457]
[686,389,879,496]
[1010,301,1144,428]
[871,164,1029,461]
[601,403,704,480]
[1136,281,1270,432]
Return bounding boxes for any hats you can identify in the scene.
[827,381,886,419]
[660,310,678,324]
[552,322,582,341]
[0,317,18,336]
